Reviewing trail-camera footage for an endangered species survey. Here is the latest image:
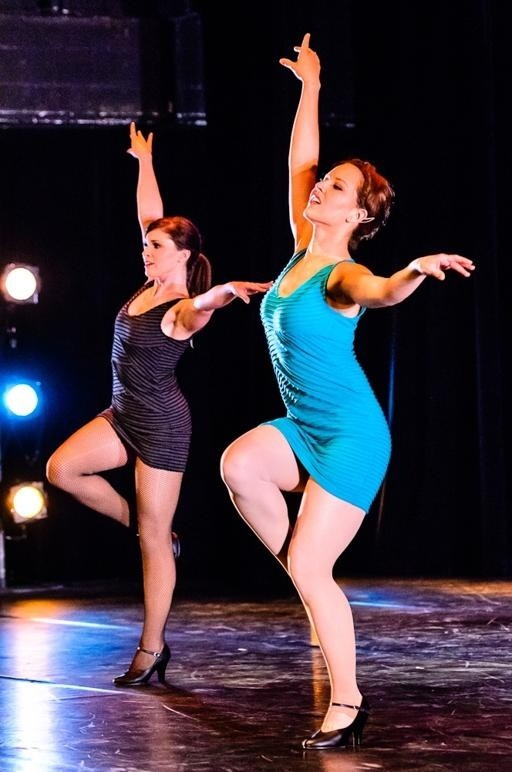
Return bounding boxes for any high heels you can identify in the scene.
[112,643,171,686]
[302,693,369,750]
[172,532,181,559]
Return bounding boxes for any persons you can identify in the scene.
[45,120,279,686]
[220,32,478,752]
